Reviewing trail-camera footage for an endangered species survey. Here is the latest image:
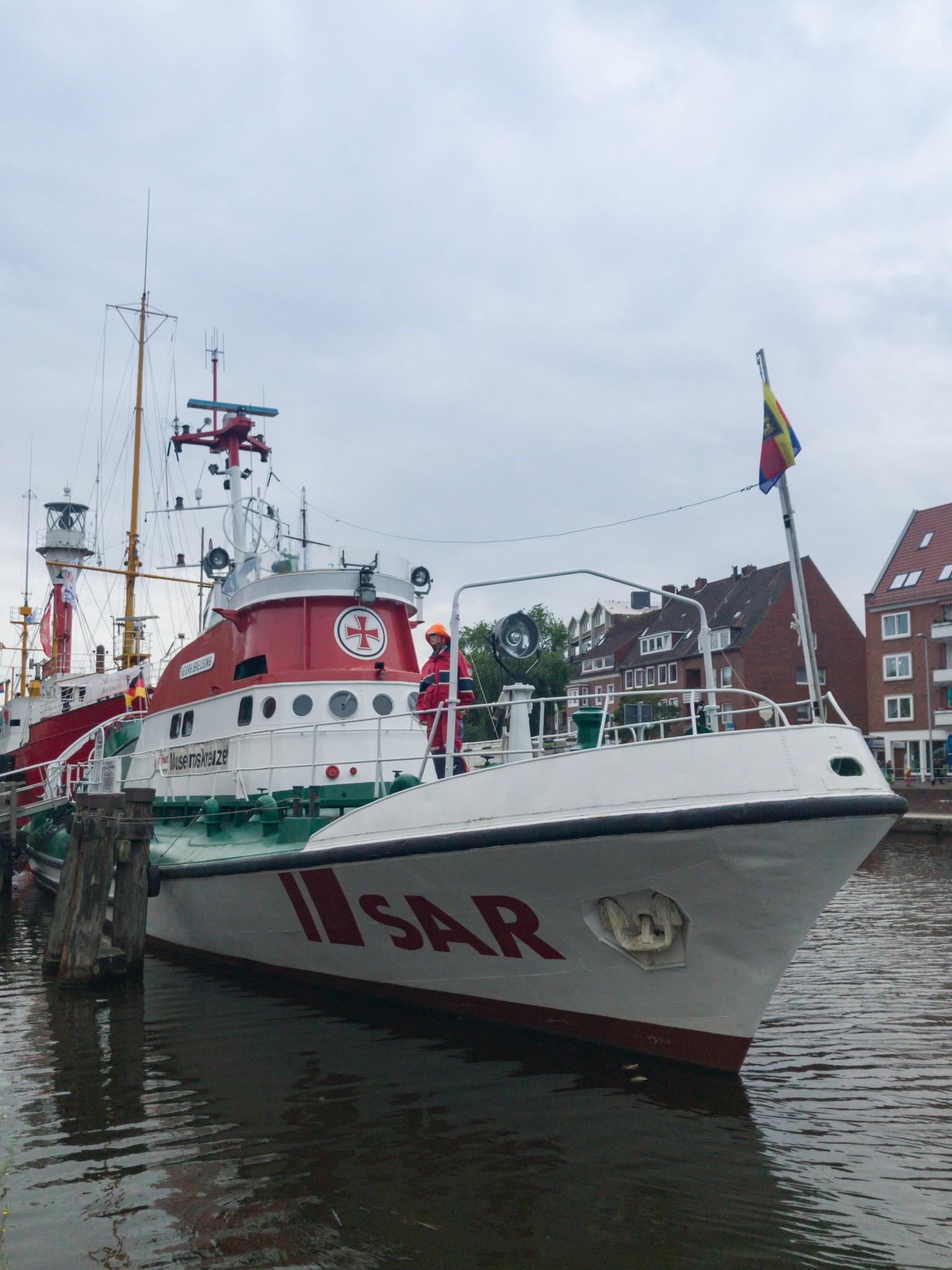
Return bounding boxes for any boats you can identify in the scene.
[19,326,910,1076]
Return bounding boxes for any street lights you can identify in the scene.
[917,633,935,785]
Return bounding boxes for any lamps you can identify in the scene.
[203,546,232,580]
[236,406,247,417]
[267,505,274,517]
[411,565,434,596]
[208,458,252,490]
[482,610,542,682]
[353,566,376,605]
[256,434,268,462]
[182,425,190,435]
[179,633,185,638]
[175,497,184,509]
[374,662,386,679]
[176,553,186,566]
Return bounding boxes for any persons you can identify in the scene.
[931,758,945,785]
[417,622,475,779]
[880,766,885,777]
[906,766,912,787]
[886,760,896,784]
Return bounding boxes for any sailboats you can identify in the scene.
[0,191,219,836]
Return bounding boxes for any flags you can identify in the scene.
[126,671,146,706]
[39,602,51,657]
[61,557,78,611]
[759,381,802,494]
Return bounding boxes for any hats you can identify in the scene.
[425,623,451,650]
[879,762,885,766]
